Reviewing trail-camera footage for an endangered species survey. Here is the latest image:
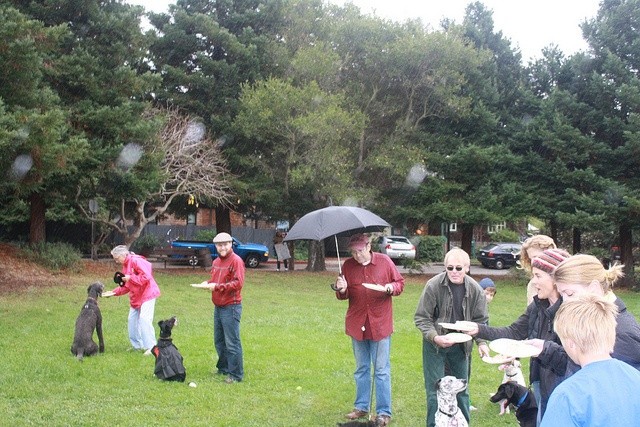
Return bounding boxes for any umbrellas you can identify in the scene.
[283,196,392,291]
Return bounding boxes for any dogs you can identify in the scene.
[490,381,539,427]
[107,323,115,324]
[70,281,114,362]
[434,375,469,427]
[490,357,526,416]
[155,314,186,383]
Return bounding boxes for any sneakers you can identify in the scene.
[225,378,240,384]
[144,349,152,355]
[126,347,134,351]
[285,268,288,271]
[346,410,367,419]
[376,415,389,426]
[276,269,280,271]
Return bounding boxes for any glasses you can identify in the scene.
[447,267,463,271]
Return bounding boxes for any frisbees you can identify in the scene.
[482,354,513,363]
[489,337,541,358]
[438,322,475,331]
[446,332,472,343]
[362,283,388,292]
[191,284,209,289]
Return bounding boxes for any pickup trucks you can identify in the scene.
[167,235,270,267]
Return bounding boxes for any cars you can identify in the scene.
[375,235,416,258]
[477,242,521,269]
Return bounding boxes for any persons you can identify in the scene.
[519,234,557,308]
[478,277,497,305]
[201,232,245,383]
[521,253,640,379]
[539,293,640,427]
[336,233,404,427]
[369,235,384,252]
[415,247,489,427]
[273,231,289,272]
[106,245,161,356]
[285,232,288,236]
[456,249,572,427]
[273,232,283,244]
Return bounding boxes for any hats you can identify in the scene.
[531,249,572,273]
[213,232,232,242]
[479,278,496,290]
[347,233,369,250]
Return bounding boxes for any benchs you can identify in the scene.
[139,247,207,271]
